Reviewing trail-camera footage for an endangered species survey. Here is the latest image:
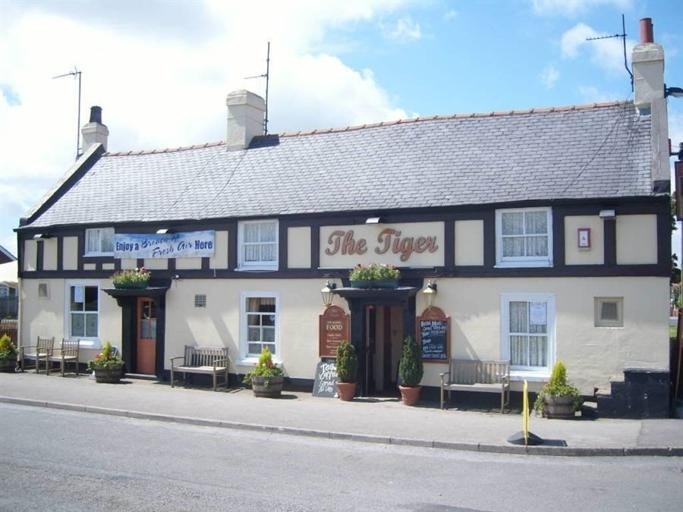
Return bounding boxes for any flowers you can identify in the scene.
[346,261,402,280]
[109,264,152,288]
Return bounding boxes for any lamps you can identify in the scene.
[420,279,440,313]
[319,280,336,308]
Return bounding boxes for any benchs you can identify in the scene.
[169,343,230,393]
[438,358,512,415]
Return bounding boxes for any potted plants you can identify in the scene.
[330,337,362,402]
[529,358,585,421]
[241,343,287,398]
[0,329,20,376]
[394,332,427,404]
[87,342,129,385]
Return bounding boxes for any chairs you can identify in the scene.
[18,336,55,376]
[42,337,80,379]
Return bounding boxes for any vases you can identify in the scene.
[348,277,399,290]
[113,281,148,289]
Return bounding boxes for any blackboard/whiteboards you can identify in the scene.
[312,359,341,398]
[415,316,450,364]
